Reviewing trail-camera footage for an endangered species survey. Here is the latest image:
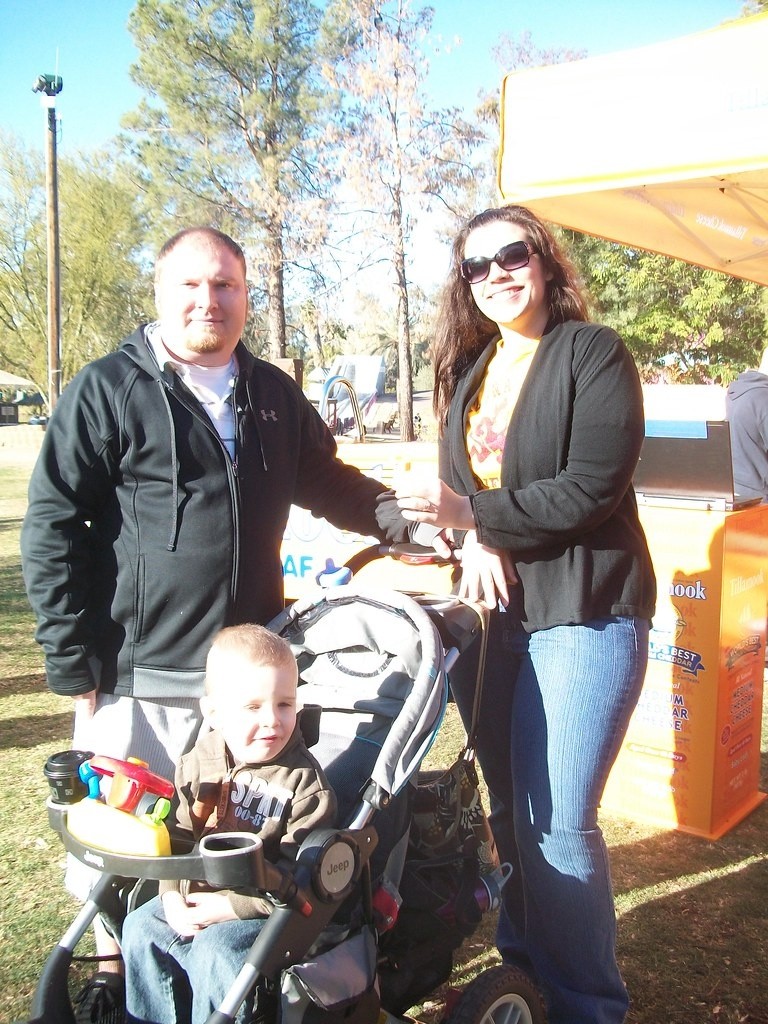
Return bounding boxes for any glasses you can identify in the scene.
[460,241,540,284]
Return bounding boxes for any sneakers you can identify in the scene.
[72,971,125,1024]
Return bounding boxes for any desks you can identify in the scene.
[597,505,768,840]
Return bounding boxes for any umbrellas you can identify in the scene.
[17,391,50,415]
[0,370,35,423]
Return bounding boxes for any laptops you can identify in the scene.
[631,421,763,513]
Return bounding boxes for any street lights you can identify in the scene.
[32,71,64,420]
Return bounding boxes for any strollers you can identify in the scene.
[18,538,550,1024]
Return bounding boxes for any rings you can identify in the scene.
[423,499,430,511]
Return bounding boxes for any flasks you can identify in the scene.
[437,862,512,927]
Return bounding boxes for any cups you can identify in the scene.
[43,750,95,803]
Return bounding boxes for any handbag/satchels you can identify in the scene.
[407,756,504,890]
[376,857,482,1017]
[275,924,380,1024]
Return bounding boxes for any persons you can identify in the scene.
[726,345,768,501]
[19,228,455,1024]
[122,624,338,1024]
[395,206,657,1024]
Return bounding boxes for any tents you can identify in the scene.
[326,355,386,400]
[498,10,768,286]
